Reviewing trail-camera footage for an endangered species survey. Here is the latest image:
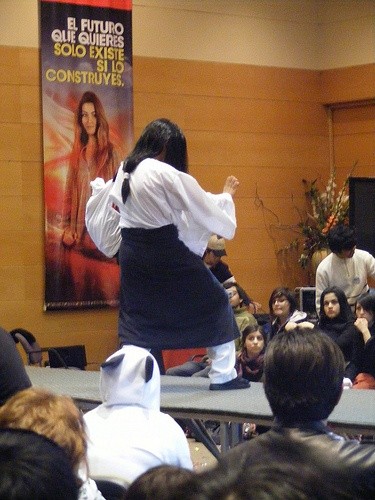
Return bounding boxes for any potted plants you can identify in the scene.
[280,159,359,285]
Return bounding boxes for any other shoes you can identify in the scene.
[209,376,250,390]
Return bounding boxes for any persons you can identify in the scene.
[313,224,374,322]
[85,117,251,391]
[163,232,258,377]
[80,344,192,499]
[311,286,356,342]
[233,323,274,442]
[334,293,375,383]
[1,385,368,499]
[262,286,319,349]
[203,325,375,499]
[56,90,123,309]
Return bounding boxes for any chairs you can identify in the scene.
[9,327,101,372]
[87,475,132,500]
